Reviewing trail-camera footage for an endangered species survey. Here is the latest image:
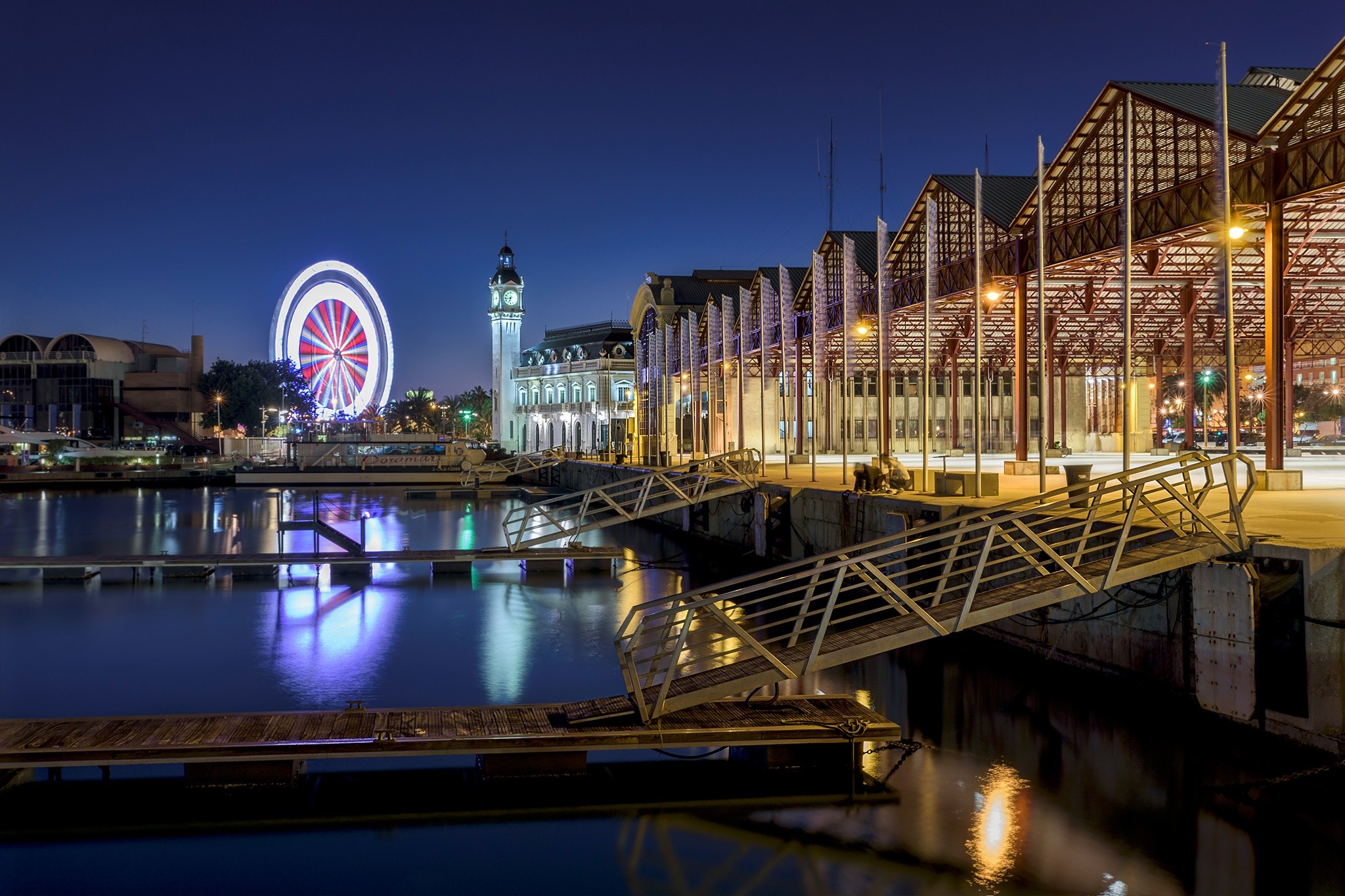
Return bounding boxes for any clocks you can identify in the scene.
[502,289,519,308]
[492,289,499,307]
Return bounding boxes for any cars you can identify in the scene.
[1309,434,1345,455]
[1159,431,1265,444]
[87,442,215,457]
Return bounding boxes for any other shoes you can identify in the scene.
[893,488,905,496]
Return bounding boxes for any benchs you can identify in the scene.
[935,471,998,496]
[907,469,943,490]
[727,459,759,474]
[643,455,672,466]
[600,453,619,461]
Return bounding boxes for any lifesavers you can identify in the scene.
[197,455,206,464]
[0,352,6,360]
[55,351,62,359]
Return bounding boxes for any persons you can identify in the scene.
[854,453,912,495]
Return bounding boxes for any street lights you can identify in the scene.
[216,397,222,456]
[465,415,470,436]
[624,388,631,456]
[259,405,278,460]
[277,406,288,433]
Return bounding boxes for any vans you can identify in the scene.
[484,439,501,453]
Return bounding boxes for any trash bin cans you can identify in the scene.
[660,451,670,467]
[1064,464,1093,508]
